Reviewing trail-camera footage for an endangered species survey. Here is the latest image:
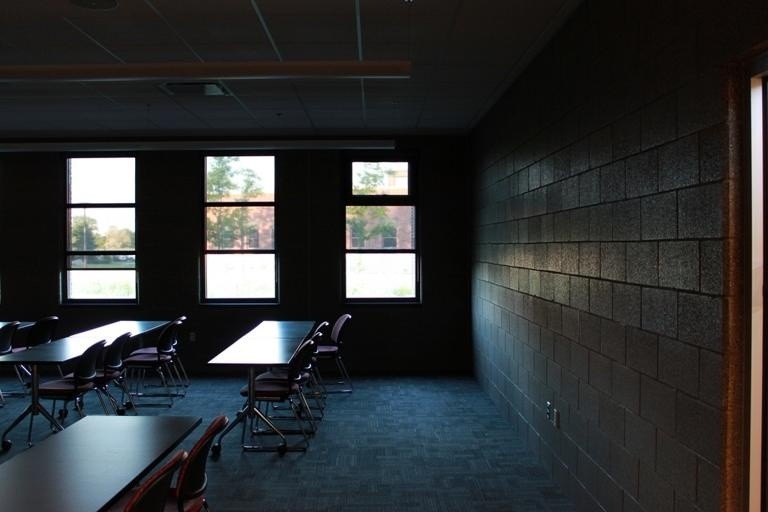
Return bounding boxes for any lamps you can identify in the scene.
[0,58,415,80]
[0,139,395,150]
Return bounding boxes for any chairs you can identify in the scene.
[130,415,229,511]
[103,449,189,512]
[268,321,329,408]
[254,330,323,433]
[240,340,315,453]
[0,307,187,450]
[317,313,354,394]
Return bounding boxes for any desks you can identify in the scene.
[206,320,316,456]
[1,414,203,511]
